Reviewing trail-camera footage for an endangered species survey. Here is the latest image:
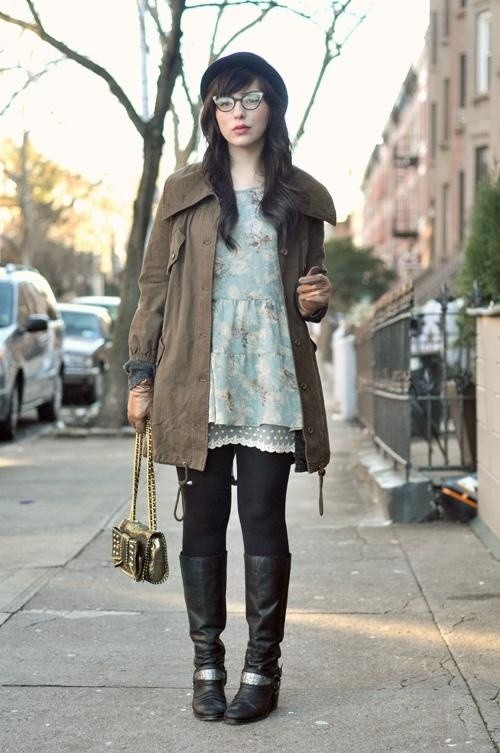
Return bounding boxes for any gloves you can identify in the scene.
[295,264,333,319]
[124,376,156,432]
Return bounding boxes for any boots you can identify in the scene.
[224,547,295,726]
[176,548,230,723]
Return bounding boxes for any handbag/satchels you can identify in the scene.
[108,420,169,585]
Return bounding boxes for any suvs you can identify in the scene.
[0,264,65,443]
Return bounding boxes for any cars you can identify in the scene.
[58,296,120,405]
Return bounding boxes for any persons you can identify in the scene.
[123,50,339,730]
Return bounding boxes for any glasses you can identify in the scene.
[211,88,266,113]
